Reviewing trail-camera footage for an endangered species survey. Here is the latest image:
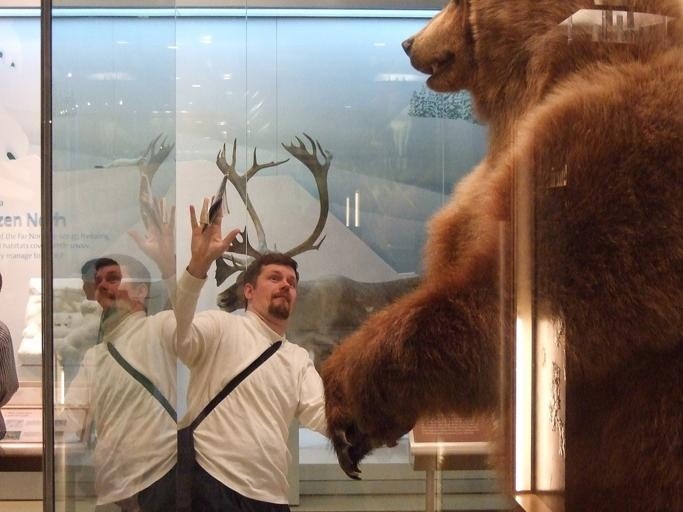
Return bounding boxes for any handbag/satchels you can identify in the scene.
[176,426,197,473]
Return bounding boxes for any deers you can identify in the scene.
[138,133,424,374]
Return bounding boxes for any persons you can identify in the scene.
[167,196,330,512]
[0,276,20,439]
[64,198,179,512]
[54,260,121,442]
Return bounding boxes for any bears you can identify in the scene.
[313,1,681,512]
